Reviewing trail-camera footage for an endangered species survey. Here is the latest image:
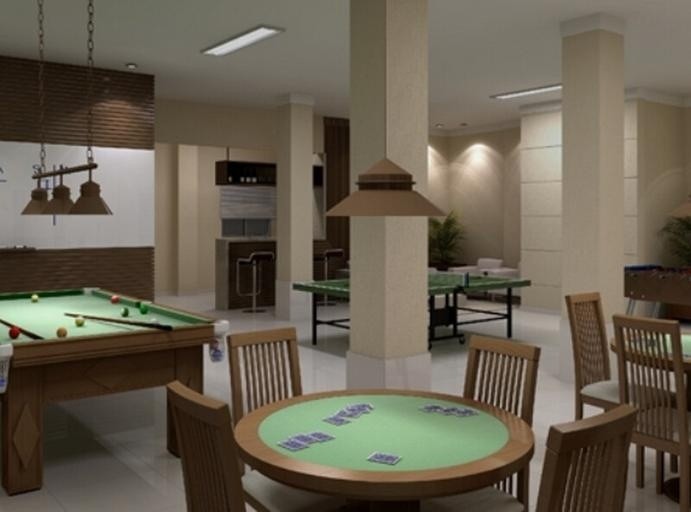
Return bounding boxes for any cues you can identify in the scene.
[0,319,45,341]
[65,313,172,331]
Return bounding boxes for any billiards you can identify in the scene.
[121,307,128,317]
[75,316,84,326]
[57,328,67,337]
[31,294,38,302]
[10,328,20,339]
[140,304,149,314]
[112,295,120,303]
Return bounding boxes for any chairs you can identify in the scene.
[315,247,345,306]
[565,292,677,488]
[167,379,257,512]
[227,327,348,512]
[448,257,520,301]
[533,402,638,512]
[234,250,276,314]
[419,336,540,512]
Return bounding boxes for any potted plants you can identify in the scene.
[428,209,469,271]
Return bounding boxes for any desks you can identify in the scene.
[293,270,531,351]
[0,287,230,496]
[231,389,535,512]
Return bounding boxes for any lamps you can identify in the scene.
[21,0,113,225]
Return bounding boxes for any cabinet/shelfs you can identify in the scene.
[214,236,277,310]
